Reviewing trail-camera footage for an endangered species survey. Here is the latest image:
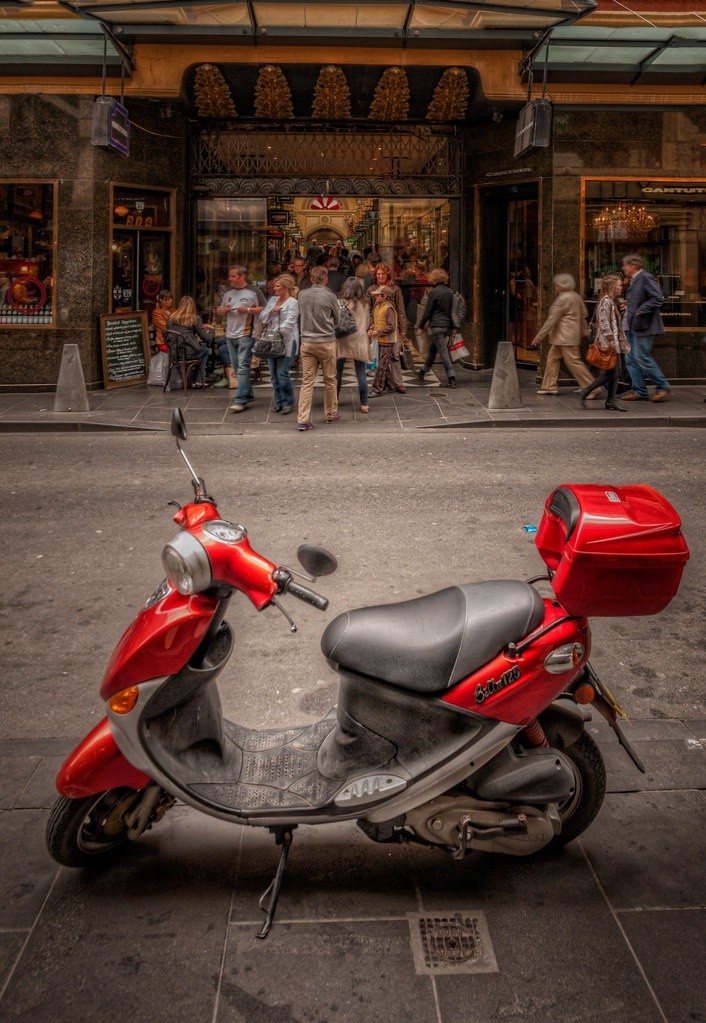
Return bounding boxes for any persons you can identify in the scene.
[620,256,672,401]
[283,239,381,297]
[284,258,312,368]
[153,290,177,352]
[335,277,369,412]
[166,296,215,387]
[441,240,449,270]
[367,285,398,397]
[297,266,341,429]
[578,274,630,412]
[212,336,230,387]
[259,274,300,412]
[414,268,457,389]
[365,263,407,392]
[531,273,602,399]
[394,245,433,274]
[216,264,267,411]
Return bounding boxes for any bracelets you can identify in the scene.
[247,308,250,313]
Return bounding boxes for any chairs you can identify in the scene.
[160,329,205,395]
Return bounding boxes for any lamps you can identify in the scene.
[591,201,656,238]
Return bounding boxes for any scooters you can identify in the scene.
[41,404,689,940]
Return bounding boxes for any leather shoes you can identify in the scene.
[651,388,671,401]
[621,391,648,400]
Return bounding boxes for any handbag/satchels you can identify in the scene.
[364,336,378,370]
[399,336,414,370]
[580,306,592,336]
[225,367,240,389]
[450,289,466,328]
[250,309,286,359]
[586,340,617,370]
[146,349,182,391]
[448,329,470,363]
[334,298,357,339]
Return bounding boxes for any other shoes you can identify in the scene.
[326,415,338,424]
[585,387,603,400]
[272,404,282,412]
[283,402,292,413]
[192,381,209,387]
[336,367,457,412]
[230,401,250,411]
[537,389,559,395]
[298,423,313,430]
[214,379,229,387]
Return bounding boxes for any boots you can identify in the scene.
[605,378,627,412]
[575,376,605,409]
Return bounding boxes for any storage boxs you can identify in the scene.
[535,483,690,617]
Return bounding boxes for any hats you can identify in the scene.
[371,285,393,297]
